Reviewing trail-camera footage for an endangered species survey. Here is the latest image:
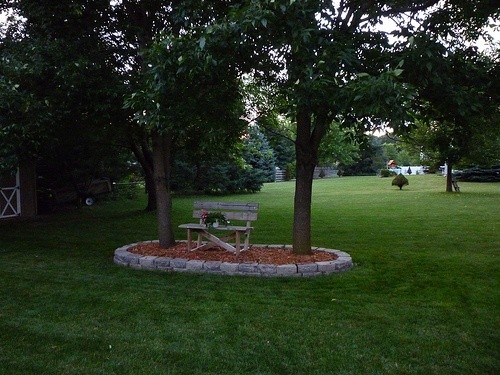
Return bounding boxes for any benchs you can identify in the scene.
[178,201,259,256]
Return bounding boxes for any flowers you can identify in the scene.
[201,209,208,222]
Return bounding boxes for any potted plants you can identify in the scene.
[205,211,227,228]
